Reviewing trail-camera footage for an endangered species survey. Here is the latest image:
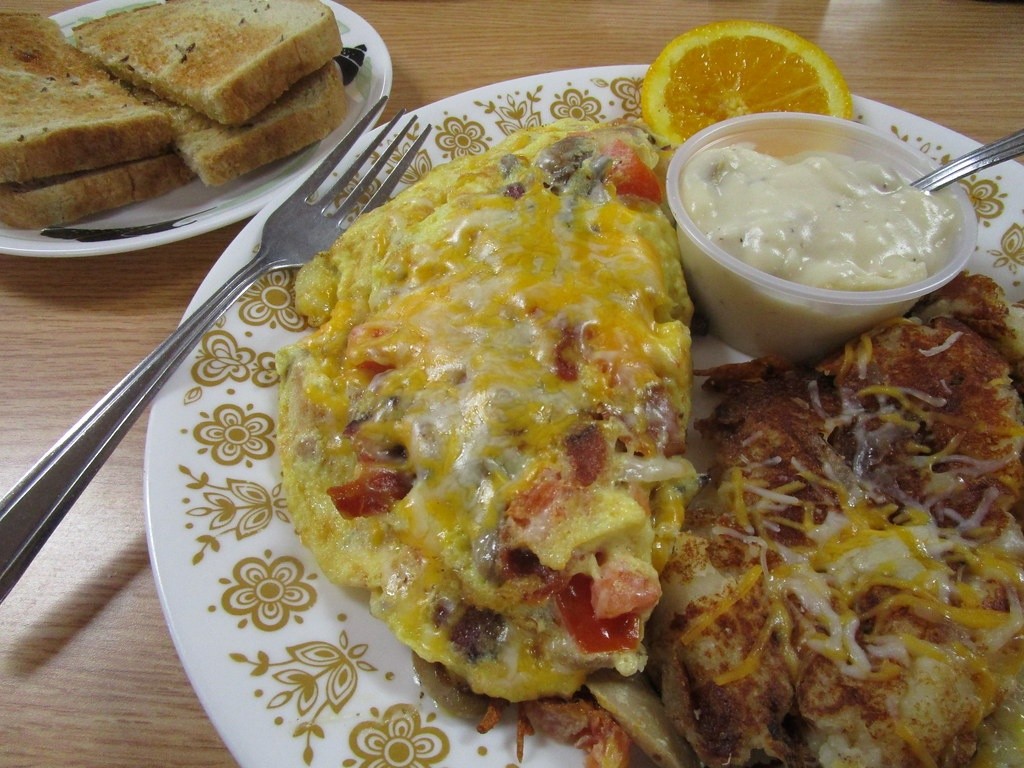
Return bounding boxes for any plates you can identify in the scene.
[144,65,1024,768]
[0,0,393,259]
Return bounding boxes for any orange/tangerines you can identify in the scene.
[639,18,852,155]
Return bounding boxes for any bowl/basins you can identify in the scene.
[665,112,978,366]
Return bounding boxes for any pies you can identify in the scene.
[519,272,1024,768]
[274,118,691,697]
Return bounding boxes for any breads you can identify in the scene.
[0,0,348,231]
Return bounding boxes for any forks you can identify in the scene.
[0,96,433,603]
